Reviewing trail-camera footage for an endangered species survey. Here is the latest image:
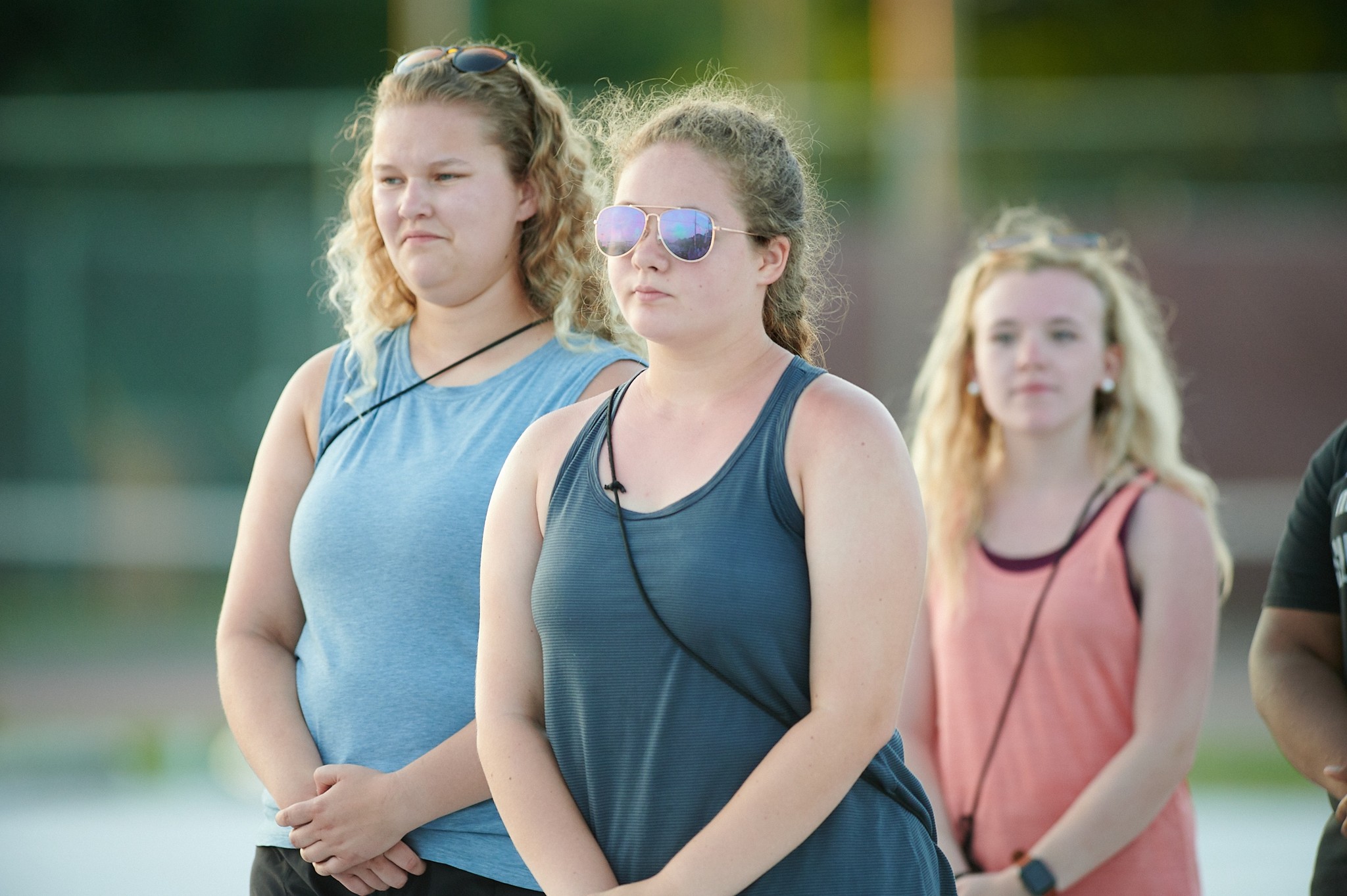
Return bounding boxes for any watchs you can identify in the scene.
[1012,849,1059,896]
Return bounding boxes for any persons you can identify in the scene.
[1246,416,1347,896]
[919,232,1220,896]
[474,101,926,896]
[214,45,648,896]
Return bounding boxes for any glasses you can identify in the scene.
[593,205,772,262]
[393,46,529,102]
